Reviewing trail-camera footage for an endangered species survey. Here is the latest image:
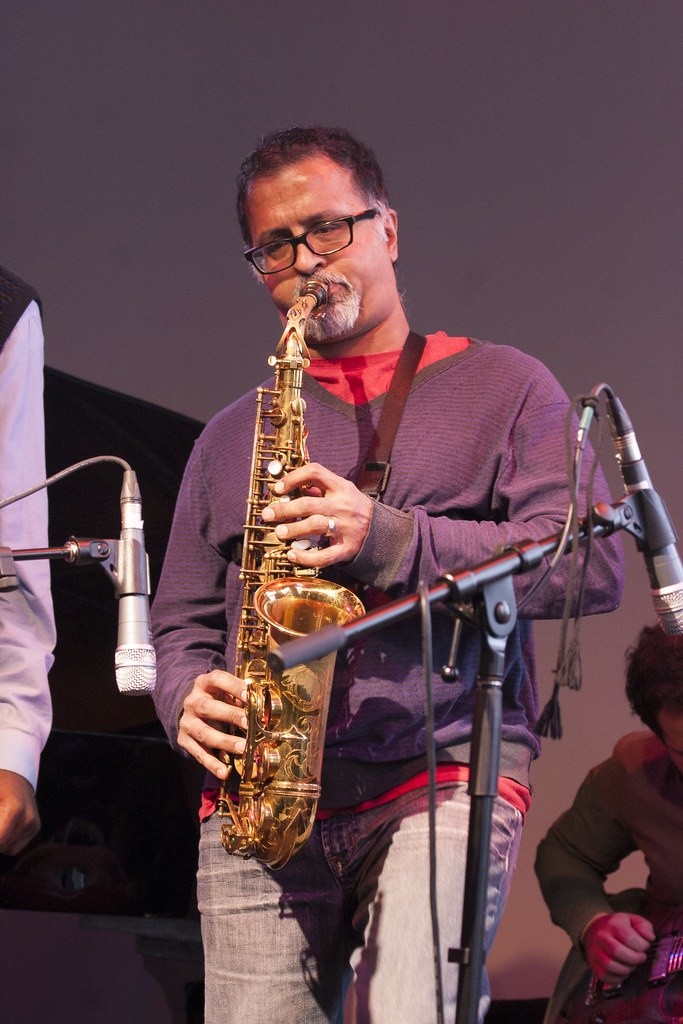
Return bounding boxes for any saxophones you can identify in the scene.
[216,280,375,866]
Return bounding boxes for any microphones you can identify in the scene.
[606,396,683,635]
[114,469,157,695]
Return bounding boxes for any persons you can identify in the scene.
[148,128,627,1024]
[1,265,59,860]
[534,624,683,984]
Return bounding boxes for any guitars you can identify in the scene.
[542,887,683,1024]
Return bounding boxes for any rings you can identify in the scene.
[327,519,336,535]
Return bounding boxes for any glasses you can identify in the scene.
[245,208,380,275]
